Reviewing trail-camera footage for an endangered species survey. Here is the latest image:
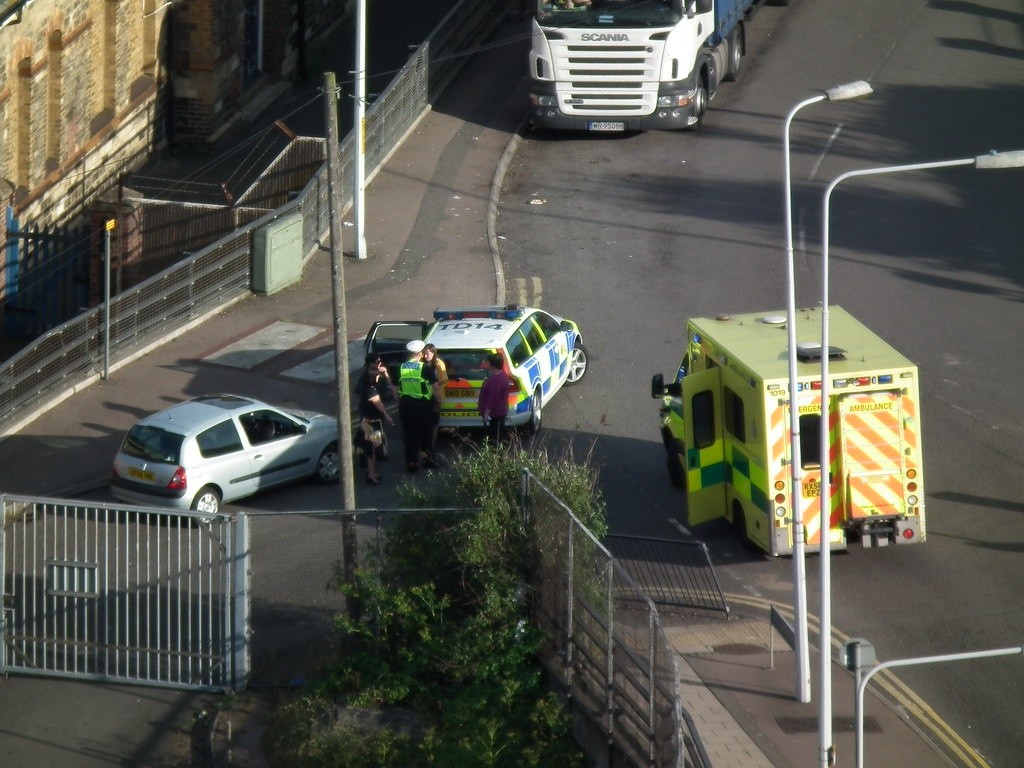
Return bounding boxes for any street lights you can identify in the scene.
[781,79,874,714]
[815,150,1024,768]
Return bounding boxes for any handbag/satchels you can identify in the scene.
[353,423,383,454]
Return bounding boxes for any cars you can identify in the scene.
[360,301,590,439]
[107,390,340,529]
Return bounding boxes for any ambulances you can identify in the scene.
[648,304,930,557]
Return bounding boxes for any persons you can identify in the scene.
[355,352,393,485]
[479,354,509,452]
[391,340,449,473]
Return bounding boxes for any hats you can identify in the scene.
[406,339,425,353]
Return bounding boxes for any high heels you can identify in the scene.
[365,474,380,485]
[366,471,382,481]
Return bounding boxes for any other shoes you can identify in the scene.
[406,466,418,474]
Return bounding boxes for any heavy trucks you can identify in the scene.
[523,0,776,130]
